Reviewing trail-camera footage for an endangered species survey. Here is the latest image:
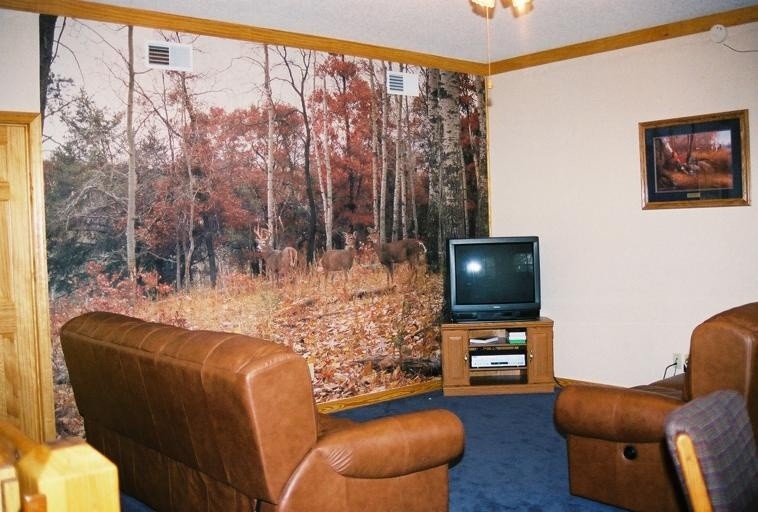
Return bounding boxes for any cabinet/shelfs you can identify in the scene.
[440,316,554,397]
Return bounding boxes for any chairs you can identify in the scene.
[664,387,758,511]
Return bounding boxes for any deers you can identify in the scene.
[367,225,427,286]
[253,221,298,275]
[320,231,358,282]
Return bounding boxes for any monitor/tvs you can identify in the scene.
[449,237,541,321]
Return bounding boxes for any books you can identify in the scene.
[506,329,527,344]
[469,336,499,343]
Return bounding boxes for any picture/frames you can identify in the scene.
[638,108,753,212]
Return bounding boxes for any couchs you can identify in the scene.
[553,301,758,512]
[58,309,463,512]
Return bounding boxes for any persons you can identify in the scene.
[672,151,693,176]
